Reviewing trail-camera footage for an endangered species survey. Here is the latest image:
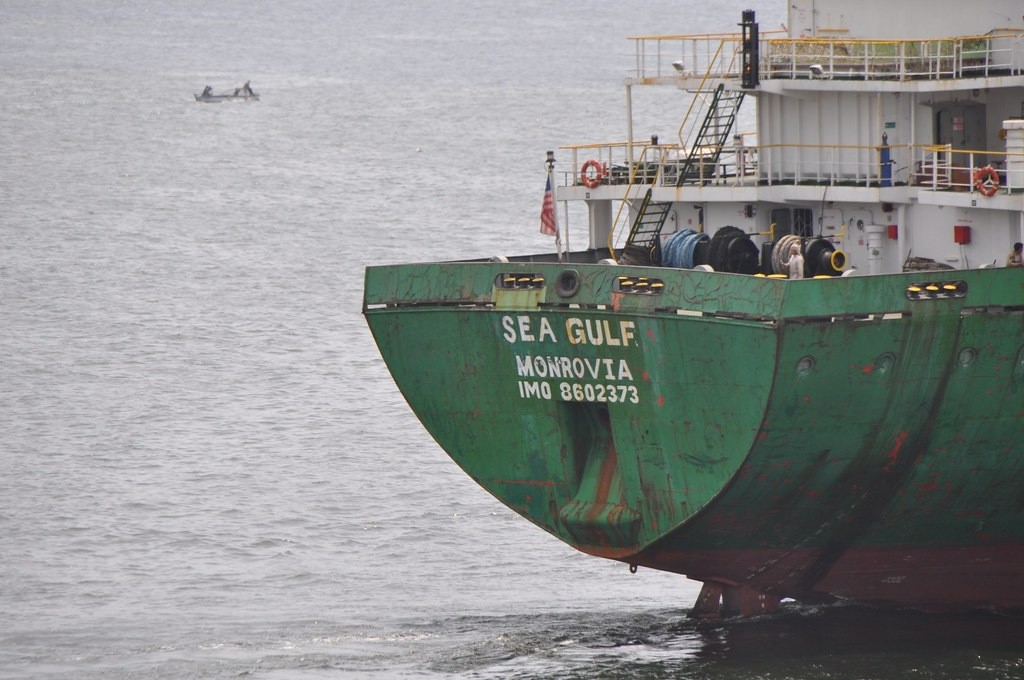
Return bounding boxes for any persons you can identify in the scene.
[1007,242,1024,266]
[202,84,214,96]
[780,243,805,283]
[245,80,255,96]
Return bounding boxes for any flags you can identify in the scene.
[539,174,558,235]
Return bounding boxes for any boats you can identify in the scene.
[355,0,1024,643]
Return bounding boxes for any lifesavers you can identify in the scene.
[581,160,602,189]
[974,166,1000,198]
